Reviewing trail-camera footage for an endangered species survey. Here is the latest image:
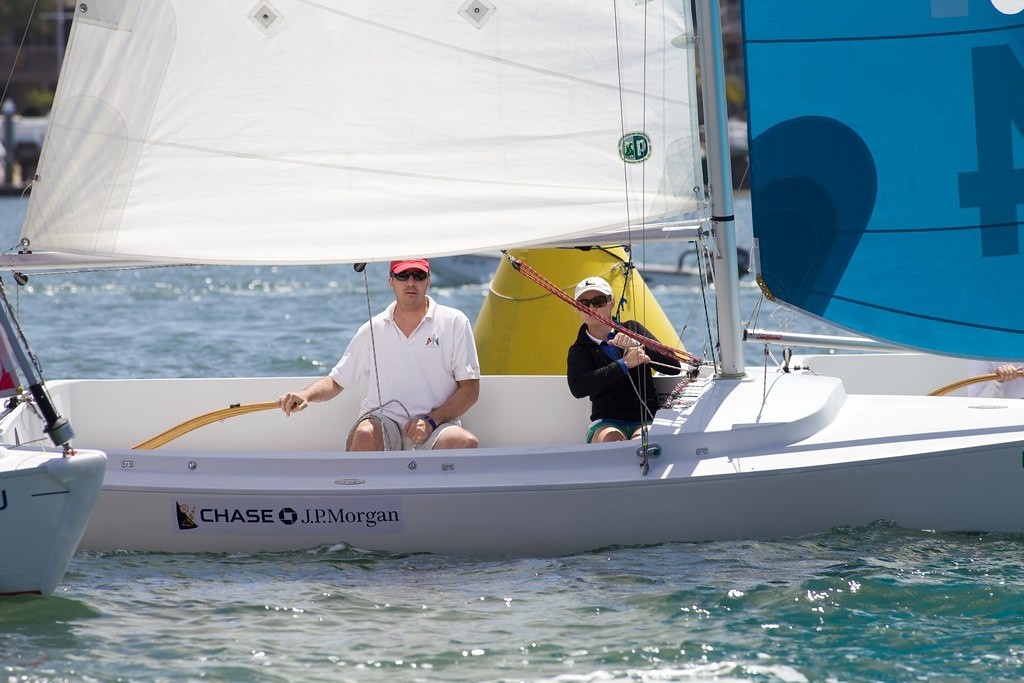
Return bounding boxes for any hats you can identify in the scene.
[574,278,613,300]
[390,260,429,274]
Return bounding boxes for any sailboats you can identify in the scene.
[0,0,1024,565]
[0,284,112,600]
[728,0,1024,403]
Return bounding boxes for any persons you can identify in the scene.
[276,258,481,452]
[567,277,681,444]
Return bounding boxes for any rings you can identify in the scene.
[414,434,417,436]
[623,341,627,345]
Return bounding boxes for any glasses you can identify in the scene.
[392,270,428,281]
[579,295,610,308]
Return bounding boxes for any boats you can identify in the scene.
[424,245,712,289]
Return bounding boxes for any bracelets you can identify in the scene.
[424,416,436,429]
